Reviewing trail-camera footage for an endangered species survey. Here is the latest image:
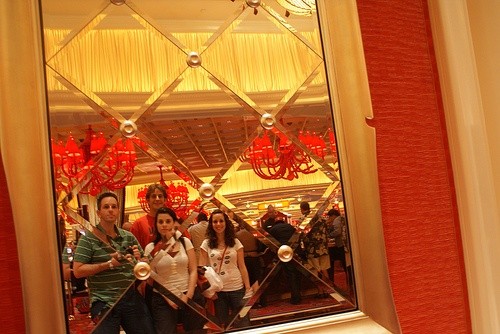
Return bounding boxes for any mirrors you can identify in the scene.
[33,0,370,334]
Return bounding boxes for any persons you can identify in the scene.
[73,193,156,334]
[238,201,347,308]
[198,209,251,328]
[131,184,192,309]
[144,207,206,334]
[62,244,71,281]
[188,212,209,286]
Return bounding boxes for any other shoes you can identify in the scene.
[323,294,328,298]
[314,293,323,299]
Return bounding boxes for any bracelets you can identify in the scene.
[108,260,114,270]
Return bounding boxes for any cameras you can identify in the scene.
[122,245,134,256]
